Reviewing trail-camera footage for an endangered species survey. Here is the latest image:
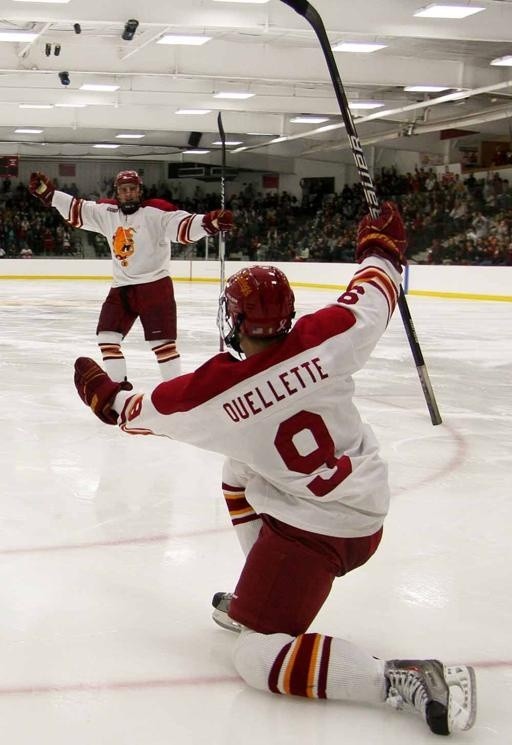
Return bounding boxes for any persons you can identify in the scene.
[0,163,511,265]
[28,169,235,391]
[73,198,450,734]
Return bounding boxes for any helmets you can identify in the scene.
[112,166,144,188]
[216,266,298,347]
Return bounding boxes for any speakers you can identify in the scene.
[188,131,202,147]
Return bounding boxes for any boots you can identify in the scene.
[380,655,450,735]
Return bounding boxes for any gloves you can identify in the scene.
[72,354,132,429]
[28,167,52,204]
[356,201,412,274]
[202,208,236,237]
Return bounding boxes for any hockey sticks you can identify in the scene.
[216,112,227,351]
[282,0,443,427]
